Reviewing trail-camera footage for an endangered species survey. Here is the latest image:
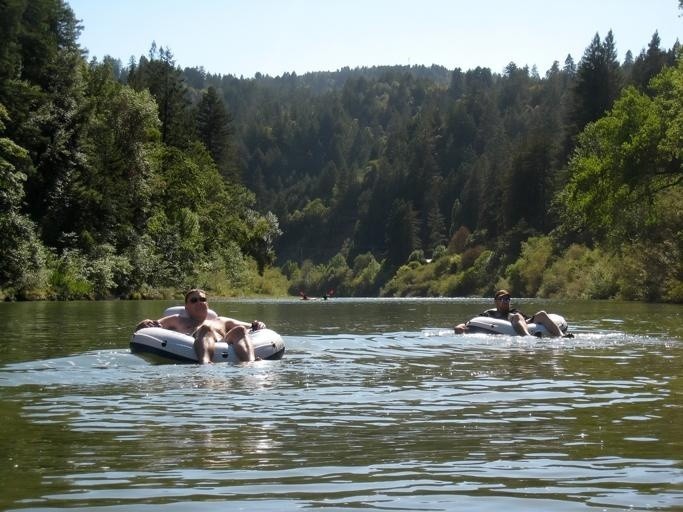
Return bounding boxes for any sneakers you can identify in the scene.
[534,331,542,337]
[562,333,575,339]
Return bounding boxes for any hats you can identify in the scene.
[495,292,511,298]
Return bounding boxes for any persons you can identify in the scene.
[133,287,267,366]
[452,288,575,340]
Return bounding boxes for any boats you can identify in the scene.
[298,297,337,300]
[127,303,288,363]
[459,312,569,341]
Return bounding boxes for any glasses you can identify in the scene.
[495,297,511,302]
[190,297,207,302]
